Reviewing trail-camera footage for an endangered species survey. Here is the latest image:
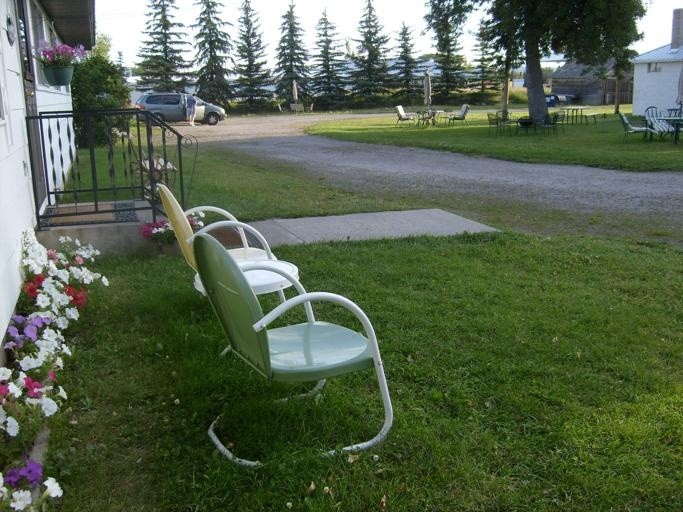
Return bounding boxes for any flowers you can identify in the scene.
[31,38,86,66]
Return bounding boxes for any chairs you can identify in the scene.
[449,105,469,128]
[278,103,314,114]
[619,111,658,141]
[155,183,299,355]
[191,232,394,468]
[395,105,416,127]
[644,106,674,140]
[487,110,565,137]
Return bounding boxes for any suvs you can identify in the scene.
[134,92,227,126]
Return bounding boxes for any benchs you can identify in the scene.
[129,140,176,194]
[583,113,605,126]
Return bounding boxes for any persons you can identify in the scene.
[186,93,197,126]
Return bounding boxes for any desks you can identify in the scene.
[561,106,588,125]
[417,110,443,126]
[193,259,299,302]
[656,117,683,143]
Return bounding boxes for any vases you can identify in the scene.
[44,66,73,86]
